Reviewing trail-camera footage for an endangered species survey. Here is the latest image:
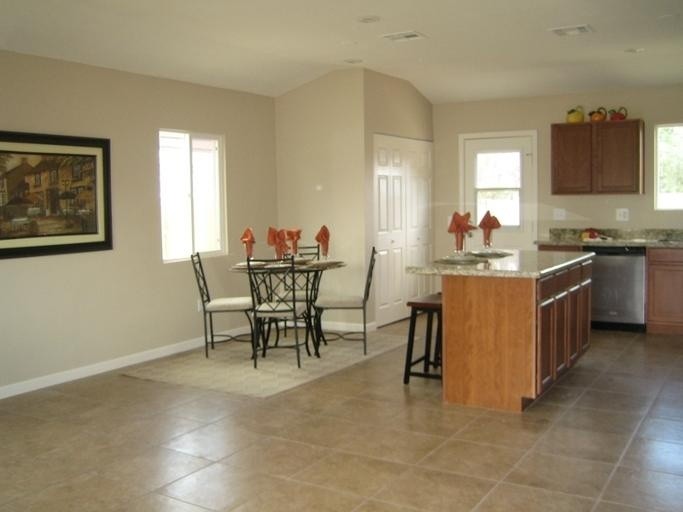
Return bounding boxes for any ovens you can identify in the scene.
[583,245,646,334]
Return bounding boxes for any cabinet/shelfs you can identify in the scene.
[443,260,594,415]
[645,247,683,336]
[551,119,645,195]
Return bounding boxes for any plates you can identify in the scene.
[311,259,344,267]
[236,261,266,266]
[281,258,312,264]
[263,264,293,269]
[469,250,513,258]
[435,256,488,265]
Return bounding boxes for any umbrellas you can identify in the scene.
[6,191,94,222]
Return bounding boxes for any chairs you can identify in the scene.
[190,243,375,369]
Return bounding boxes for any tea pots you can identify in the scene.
[607,106,628,120]
[565,105,584,122]
[588,106,608,121]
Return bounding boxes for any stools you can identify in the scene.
[404,293,443,386]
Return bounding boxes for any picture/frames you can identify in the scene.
[0,130,113,260]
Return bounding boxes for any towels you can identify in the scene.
[478,210,501,248]
[314,225,329,256]
[447,212,477,251]
[268,226,302,260]
[240,227,256,257]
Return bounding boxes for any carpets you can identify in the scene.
[119,326,420,400]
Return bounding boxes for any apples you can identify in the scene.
[588,231,598,238]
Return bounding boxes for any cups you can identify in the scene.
[274,247,283,263]
[319,241,327,260]
[482,228,491,247]
[454,232,463,253]
[245,243,252,259]
[291,240,297,255]
